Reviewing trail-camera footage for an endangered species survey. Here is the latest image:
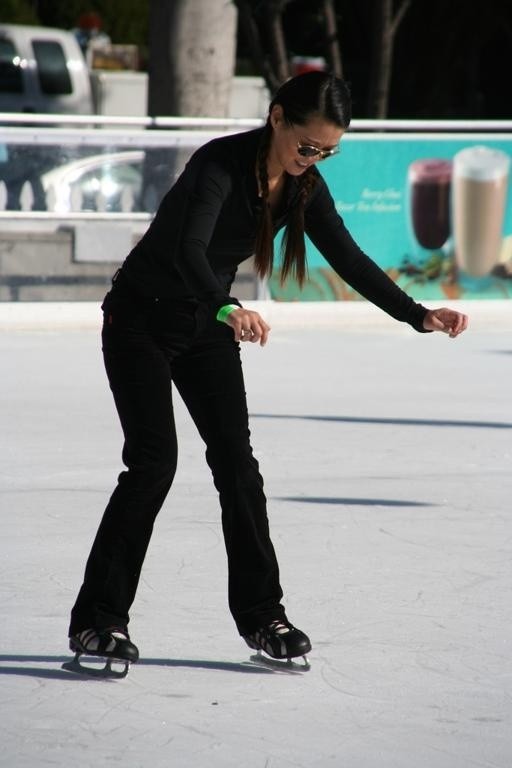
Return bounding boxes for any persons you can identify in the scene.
[68,71,468,660]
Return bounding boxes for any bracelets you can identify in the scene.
[216,303,239,323]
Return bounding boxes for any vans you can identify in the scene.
[1,23,96,131]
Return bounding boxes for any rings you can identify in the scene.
[244,329,253,338]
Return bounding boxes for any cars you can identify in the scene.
[2,151,147,213]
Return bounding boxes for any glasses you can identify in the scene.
[284,115,341,162]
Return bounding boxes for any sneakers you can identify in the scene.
[69,625,139,662]
[242,615,311,659]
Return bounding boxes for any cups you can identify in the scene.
[409,145,508,291]
